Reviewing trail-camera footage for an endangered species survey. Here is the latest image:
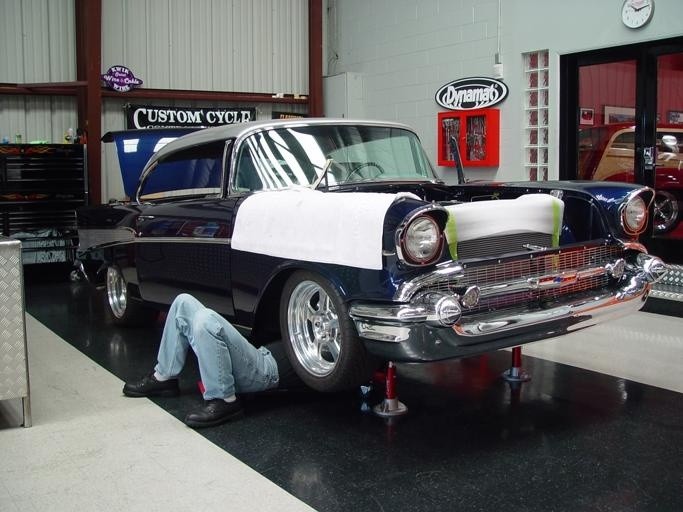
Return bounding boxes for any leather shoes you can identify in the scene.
[185,396,245,428]
[123,372,180,398]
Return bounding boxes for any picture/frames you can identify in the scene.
[577,104,682,126]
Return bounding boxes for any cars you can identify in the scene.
[68,110,659,413]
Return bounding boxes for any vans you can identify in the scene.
[573,114,682,237]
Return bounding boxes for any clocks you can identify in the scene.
[620,1,655,30]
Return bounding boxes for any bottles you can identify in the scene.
[15,134,22,145]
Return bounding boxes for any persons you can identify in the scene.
[116,289,319,429]
